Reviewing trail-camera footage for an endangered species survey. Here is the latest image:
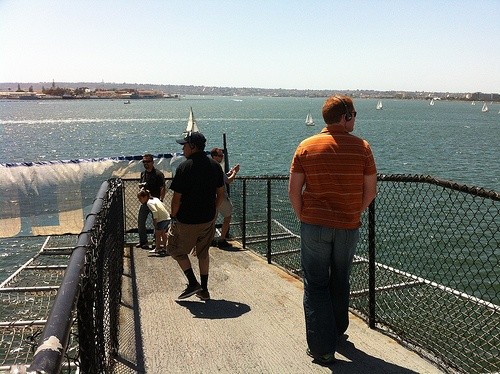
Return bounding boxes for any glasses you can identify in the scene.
[349,112,357,118]
[214,154,224,157]
[142,160,152,164]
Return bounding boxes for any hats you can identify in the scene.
[175,132,206,149]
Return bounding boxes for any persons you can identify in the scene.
[137,190,171,253]
[166,131,224,300]
[210,147,240,247]
[136,154,166,248]
[288,95,377,363]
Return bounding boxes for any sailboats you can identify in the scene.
[430,99,434,106]
[304,111,315,126]
[481,103,488,112]
[184,107,200,138]
[376,101,383,110]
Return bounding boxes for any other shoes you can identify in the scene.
[177,281,203,299]
[218,238,232,247]
[195,288,210,300]
[135,242,148,248]
[306,347,332,366]
